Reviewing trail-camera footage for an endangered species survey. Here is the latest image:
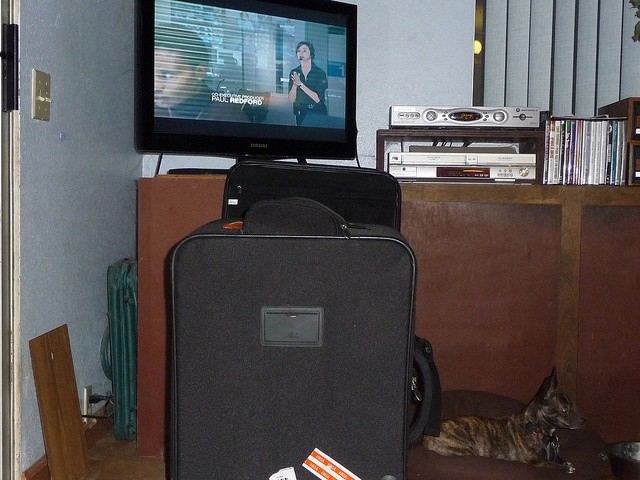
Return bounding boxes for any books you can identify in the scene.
[543,120,627,186]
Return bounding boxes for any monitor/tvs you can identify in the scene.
[133,0,357,175]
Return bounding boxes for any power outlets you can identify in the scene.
[84,386,93,426]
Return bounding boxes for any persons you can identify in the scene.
[154,22,251,124]
[288,42,330,127]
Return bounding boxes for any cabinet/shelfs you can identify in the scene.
[377,97,640,186]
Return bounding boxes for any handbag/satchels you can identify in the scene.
[222,160,402,231]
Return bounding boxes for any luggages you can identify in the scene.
[164,196,442,479]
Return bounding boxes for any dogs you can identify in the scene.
[424,367,585,474]
